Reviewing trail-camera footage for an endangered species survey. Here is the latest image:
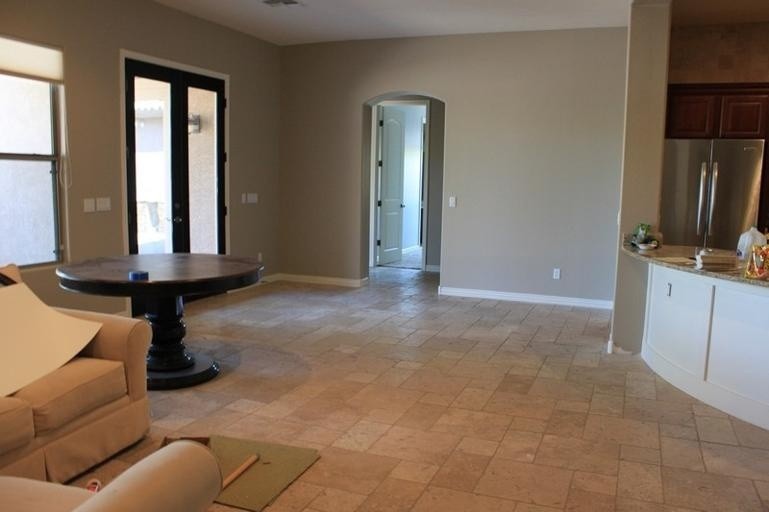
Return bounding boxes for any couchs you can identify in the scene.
[0,262,155,485]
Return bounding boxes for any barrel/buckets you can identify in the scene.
[735,222,767,265]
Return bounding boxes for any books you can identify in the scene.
[696,253,738,272]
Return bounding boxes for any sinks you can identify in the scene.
[689,255,738,263]
[704,262,737,274]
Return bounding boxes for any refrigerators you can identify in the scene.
[657,137,765,251]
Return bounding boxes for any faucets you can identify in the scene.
[693,246,711,271]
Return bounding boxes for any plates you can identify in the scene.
[637,243,658,250]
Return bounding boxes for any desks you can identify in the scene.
[54,252,265,391]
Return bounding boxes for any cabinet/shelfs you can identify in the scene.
[641,263,769,432]
[664,82,769,140]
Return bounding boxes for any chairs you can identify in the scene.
[0,438,223,512]
